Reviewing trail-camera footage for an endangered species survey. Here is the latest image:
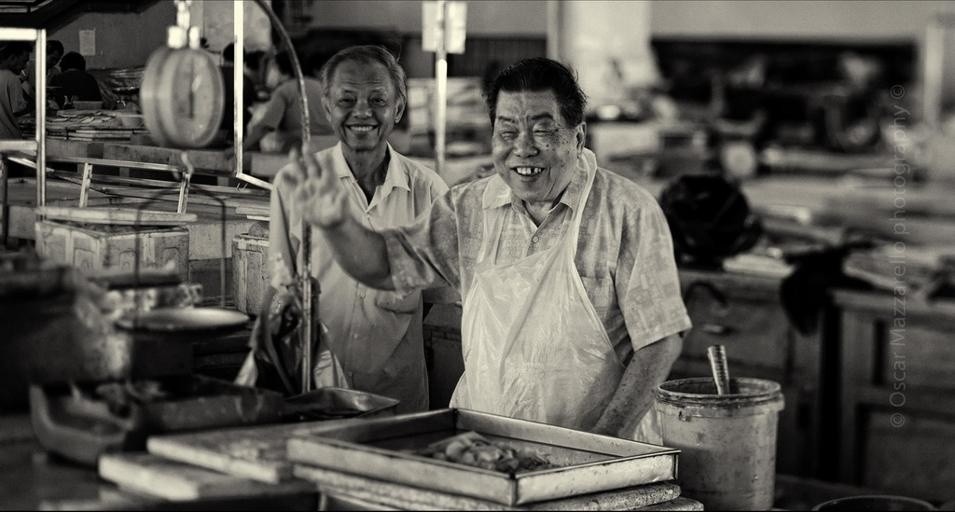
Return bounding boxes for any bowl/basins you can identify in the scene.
[119,115,142,128]
[811,493,937,510]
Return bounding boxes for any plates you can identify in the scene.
[110,305,257,338]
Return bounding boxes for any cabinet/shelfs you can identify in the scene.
[661,269,955,506]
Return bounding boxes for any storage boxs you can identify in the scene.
[31,204,199,285]
[228,203,271,317]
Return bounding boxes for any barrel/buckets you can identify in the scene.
[654,376,786,511]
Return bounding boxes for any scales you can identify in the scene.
[110,0,251,339]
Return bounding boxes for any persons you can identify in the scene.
[0,41,30,139]
[268,45,449,415]
[20,38,63,90]
[50,52,99,108]
[281,57,693,439]
